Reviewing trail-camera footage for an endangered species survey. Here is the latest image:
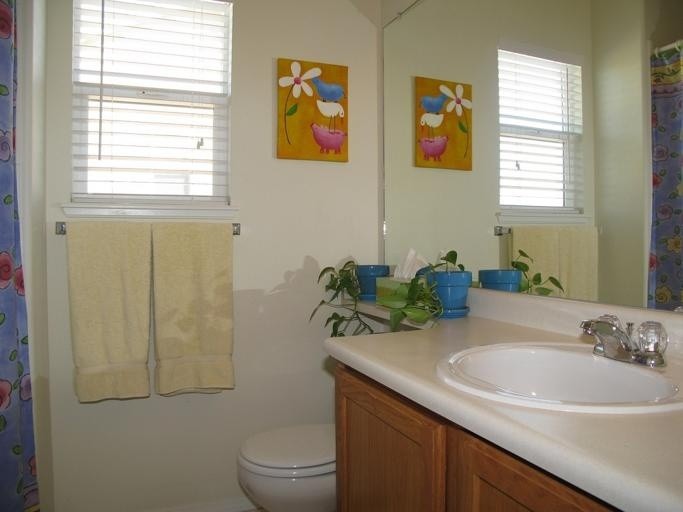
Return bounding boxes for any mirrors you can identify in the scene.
[384,1,682,313]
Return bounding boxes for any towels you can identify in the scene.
[151,220,235,397]
[508,223,559,297]
[65,220,152,403]
[559,224,598,301]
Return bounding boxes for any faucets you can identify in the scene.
[579,315,668,367]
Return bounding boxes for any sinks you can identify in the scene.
[436,341,683,416]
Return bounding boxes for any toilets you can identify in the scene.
[236,423,337,511]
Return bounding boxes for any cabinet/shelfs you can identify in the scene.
[448,429,592,512]
[336,365,446,512]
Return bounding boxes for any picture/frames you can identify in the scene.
[277,58,348,162]
[414,75,472,172]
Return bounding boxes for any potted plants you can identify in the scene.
[390,250,472,333]
[310,260,389,337]
[478,249,565,296]
